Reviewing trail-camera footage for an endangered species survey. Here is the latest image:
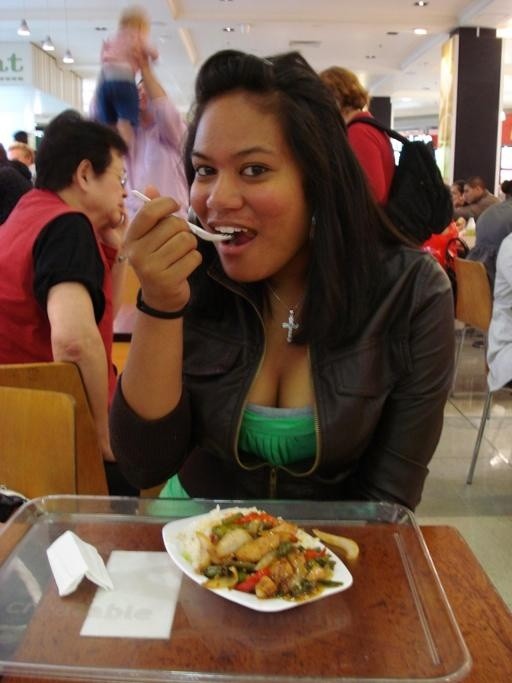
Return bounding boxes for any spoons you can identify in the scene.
[130,188,233,242]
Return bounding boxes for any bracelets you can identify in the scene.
[103,250,130,264]
[134,286,192,319]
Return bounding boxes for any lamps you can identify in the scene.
[42,0,56,51]
[63,8,74,64]
[17,1,31,36]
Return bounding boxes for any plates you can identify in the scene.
[162,505,354,614]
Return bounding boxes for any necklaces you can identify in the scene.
[261,282,312,346]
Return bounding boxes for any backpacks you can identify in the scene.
[377,137,454,253]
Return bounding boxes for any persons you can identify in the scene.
[323,63,395,210]
[462,179,512,277]
[91,10,159,144]
[110,49,453,526]
[450,173,467,203]
[95,39,190,217]
[3,141,33,202]
[483,224,512,393]
[451,177,500,219]
[0,111,168,518]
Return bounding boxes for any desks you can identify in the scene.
[0,512,512,683]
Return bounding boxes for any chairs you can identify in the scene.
[467,360,512,483]
[448,257,494,420]
[1,386,79,582]
[1,362,115,515]
